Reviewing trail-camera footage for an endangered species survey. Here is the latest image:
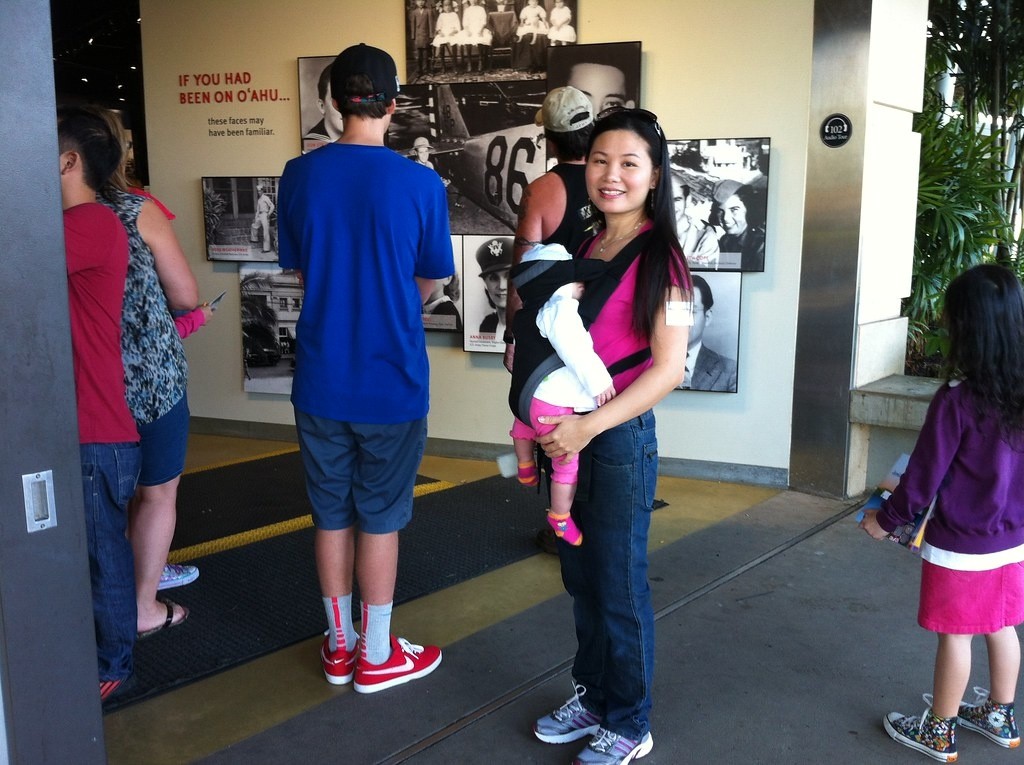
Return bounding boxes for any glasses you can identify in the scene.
[597,106,662,140]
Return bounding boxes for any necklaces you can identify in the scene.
[599,216,648,253]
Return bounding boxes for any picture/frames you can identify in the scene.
[201,175,280,263]
[296,56,344,155]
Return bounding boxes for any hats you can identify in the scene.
[476,238,515,277]
[409,137,434,156]
[712,180,742,203]
[534,86,593,133]
[257,184,263,190]
[330,43,400,103]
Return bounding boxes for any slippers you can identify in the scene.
[134,600,190,639]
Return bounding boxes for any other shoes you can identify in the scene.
[98,679,124,701]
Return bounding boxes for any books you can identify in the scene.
[856,450,937,554]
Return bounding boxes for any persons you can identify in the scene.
[477,237,515,344]
[667,166,766,273]
[57,114,144,700]
[855,264,1023,765]
[275,44,458,696]
[419,267,462,330]
[250,184,274,253]
[531,109,693,765]
[677,275,738,394]
[410,136,436,170]
[94,111,217,637]
[411,0,578,73]
[508,243,616,547]
[503,84,606,555]
[566,44,636,120]
[303,62,345,143]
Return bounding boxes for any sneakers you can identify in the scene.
[572,727,653,765]
[532,680,605,744]
[355,632,442,694]
[883,694,957,763]
[157,561,200,592]
[320,625,361,686]
[957,687,1020,748]
